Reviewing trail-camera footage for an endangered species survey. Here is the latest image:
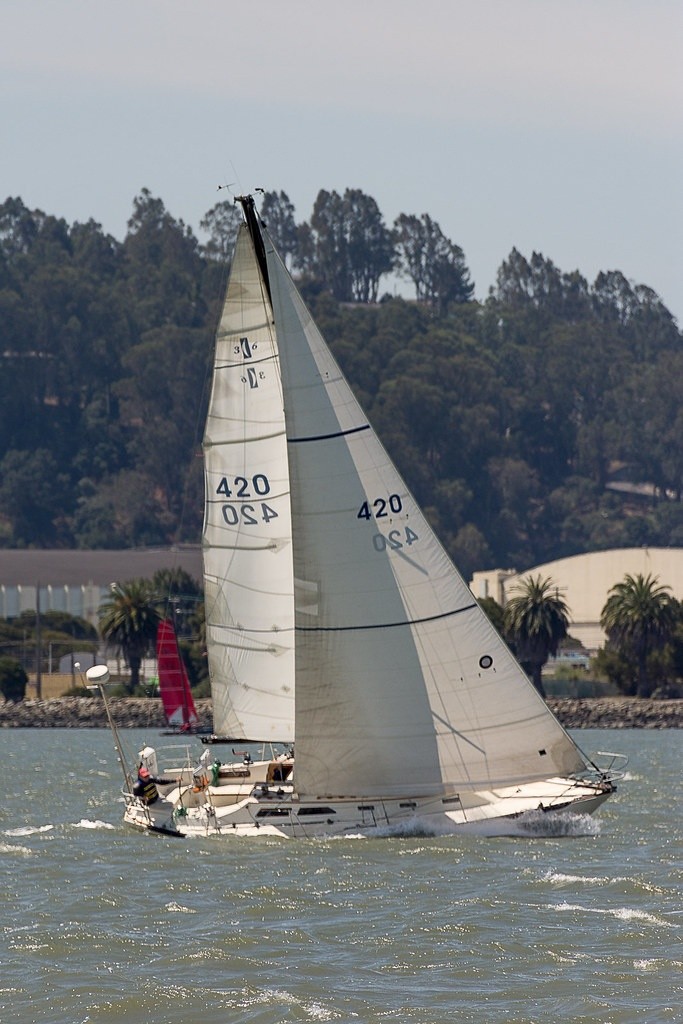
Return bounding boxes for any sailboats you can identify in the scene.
[124,185,617,840]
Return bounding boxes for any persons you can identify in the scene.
[131,767,183,810]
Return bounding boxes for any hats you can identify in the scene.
[138,767,151,778]
